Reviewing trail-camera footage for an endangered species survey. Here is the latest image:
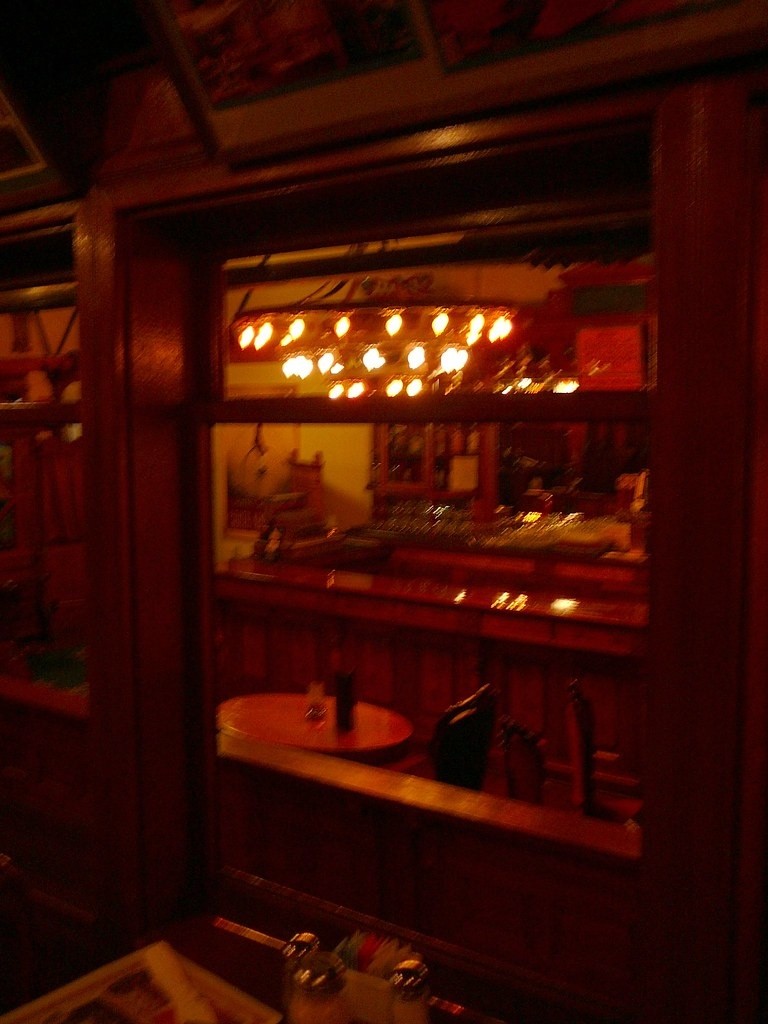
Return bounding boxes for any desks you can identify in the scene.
[215,691,414,760]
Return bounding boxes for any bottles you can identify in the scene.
[335,671,355,733]
[305,681,328,724]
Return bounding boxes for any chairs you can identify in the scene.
[370,678,647,822]
